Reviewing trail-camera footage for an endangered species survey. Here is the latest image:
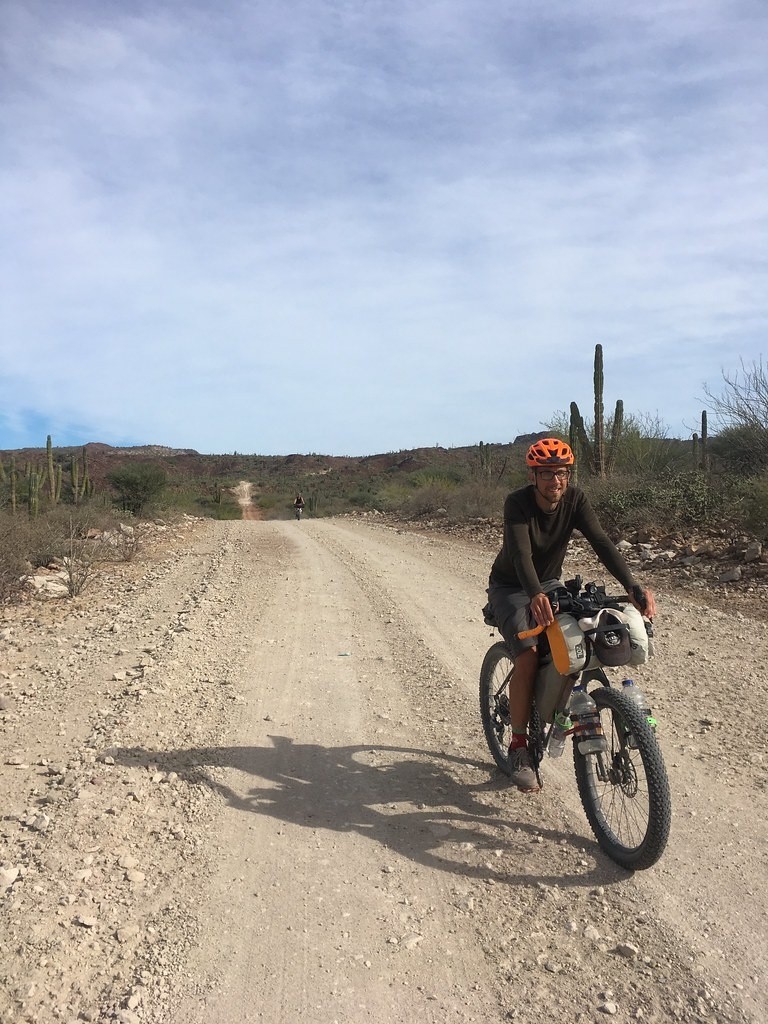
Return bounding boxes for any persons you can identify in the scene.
[293,492,305,516]
[488,439,657,790]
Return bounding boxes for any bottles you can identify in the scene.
[548,709,569,758]
[570,683,605,755]
[620,677,655,748]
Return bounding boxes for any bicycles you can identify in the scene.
[478,574,673,870]
[294,503,304,521]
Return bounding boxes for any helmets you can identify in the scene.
[525,439,576,466]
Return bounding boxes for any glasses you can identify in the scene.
[533,471,572,480]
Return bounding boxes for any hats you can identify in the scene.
[579,608,631,667]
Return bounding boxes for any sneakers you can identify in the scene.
[507,744,540,788]
